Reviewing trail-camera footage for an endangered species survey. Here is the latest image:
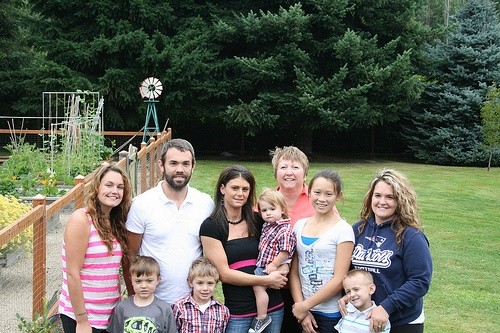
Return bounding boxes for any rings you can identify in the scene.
[298,320,301,322]
[378,326,381,328]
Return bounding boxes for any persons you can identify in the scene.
[106,256,179,333]
[342,169,433,333]
[253,146,341,227]
[57,164,132,333]
[124,139,216,310]
[170,257,230,333]
[198,165,292,333]
[248,189,297,333]
[333,269,391,333]
[289,170,356,333]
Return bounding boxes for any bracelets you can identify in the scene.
[74,312,87,316]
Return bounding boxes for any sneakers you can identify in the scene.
[248,316,271,333]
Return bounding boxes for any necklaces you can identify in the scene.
[227,216,244,224]
